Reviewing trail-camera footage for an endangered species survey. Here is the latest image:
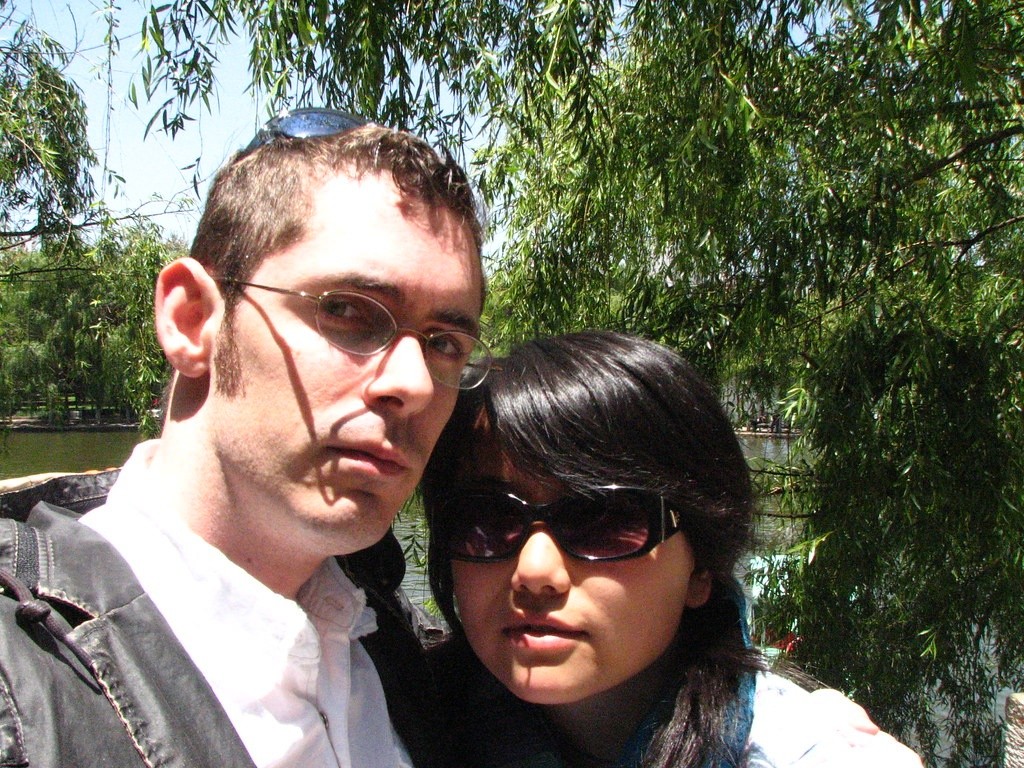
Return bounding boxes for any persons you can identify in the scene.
[393,330,925,768]
[746,404,781,433]
[0,108,492,768]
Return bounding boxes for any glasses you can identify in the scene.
[428,485,684,565]
[231,107,363,165]
[212,275,503,390]
